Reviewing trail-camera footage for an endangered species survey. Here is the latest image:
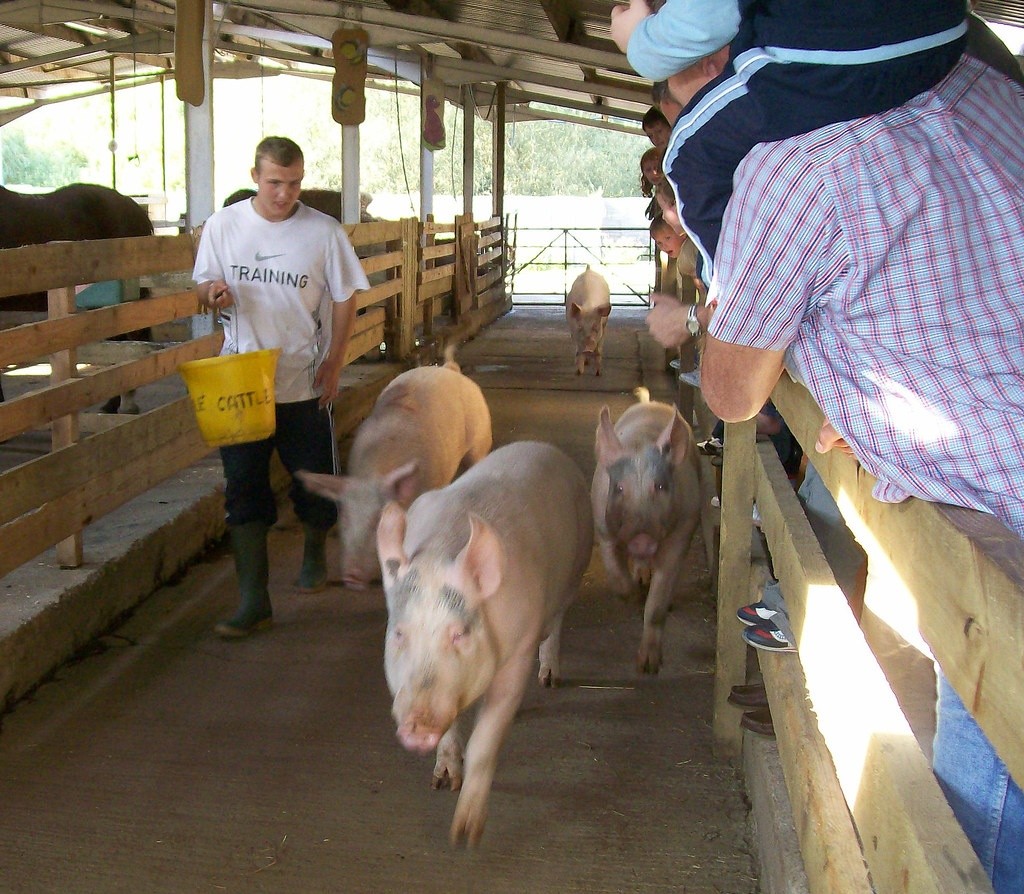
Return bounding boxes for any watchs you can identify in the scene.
[684,302,702,336]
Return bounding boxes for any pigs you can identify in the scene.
[565,263,613,378]
[587,384,704,677]
[374,441,587,847]
[293,343,494,592]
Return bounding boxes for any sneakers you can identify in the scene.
[679,368,701,387]
[736,600,799,653]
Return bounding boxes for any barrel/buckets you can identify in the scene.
[179,294,281,447]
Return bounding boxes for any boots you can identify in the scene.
[300,518,327,594]
[216,520,272,637]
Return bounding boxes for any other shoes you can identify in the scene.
[697,437,725,454]
[728,684,776,741]
[711,496,721,507]
[669,359,681,369]
[711,457,722,466]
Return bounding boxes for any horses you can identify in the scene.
[0,184,155,417]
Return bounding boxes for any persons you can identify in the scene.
[650,77,683,128]
[738,458,870,653]
[710,397,793,530]
[654,175,686,236]
[649,211,703,387]
[727,555,870,737]
[677,237,724,467]
[641,0,1024,893]
[642,105,670,146]
[640,146,666,198]
[608,0,969,294]
[190,134,372,637]
